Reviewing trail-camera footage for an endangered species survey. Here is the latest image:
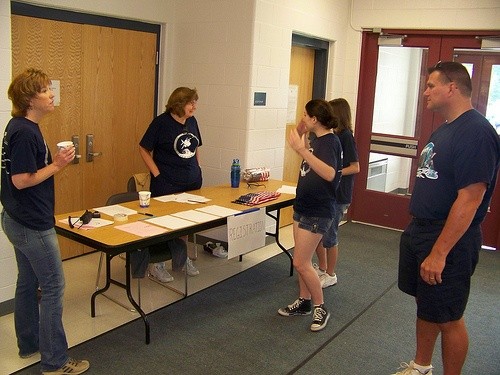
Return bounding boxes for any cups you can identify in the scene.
[56,141,73,154]
[138,191,151,208]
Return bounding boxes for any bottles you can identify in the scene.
[230,159,240,188]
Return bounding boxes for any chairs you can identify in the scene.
[118,175,198,262]
[93,192,187,314]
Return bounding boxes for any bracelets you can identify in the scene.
[295,147,306,154]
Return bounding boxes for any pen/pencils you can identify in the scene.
[188,199,206,204]
[138,212,153,216]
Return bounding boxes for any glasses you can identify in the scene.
[435,60,458,90]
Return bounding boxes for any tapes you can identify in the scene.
[113,213,129,221]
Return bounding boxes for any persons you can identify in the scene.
[313,98,360,289]
[0,68,89,375]
[397,62,500,375]
[147,86,203,283]
[278,100,343,331]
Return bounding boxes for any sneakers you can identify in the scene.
[310,304,331,331]
[313,263,326,276]
[278,297,312,316]
[390,361,434,375]
[320,273,337,288]
[42,358,90,375]
[148,262,174,283]
[182,257,199,276]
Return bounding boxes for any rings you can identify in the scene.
[430,278,434,279]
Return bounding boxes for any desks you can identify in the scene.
[53,179,298,344]
